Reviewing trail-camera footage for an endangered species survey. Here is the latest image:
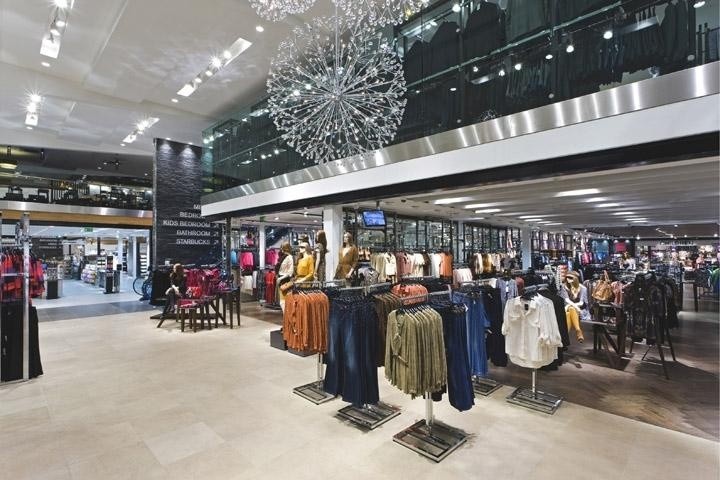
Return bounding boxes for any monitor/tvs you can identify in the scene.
[362,210,387,226]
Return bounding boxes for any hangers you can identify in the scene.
[291,270,563,328]
[634,269,669,296]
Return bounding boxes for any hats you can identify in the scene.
[566,271,578,279]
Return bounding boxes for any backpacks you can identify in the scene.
[185,286,202,299]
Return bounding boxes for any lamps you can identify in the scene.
[249,1,430,166]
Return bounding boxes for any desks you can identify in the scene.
[578,319,622,367]
[591,303,626,368]
[179,295,220,333]
[215,287,241,328]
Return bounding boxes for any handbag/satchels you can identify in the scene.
[592,270,613,302]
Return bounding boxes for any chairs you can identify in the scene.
[0,182,152,210]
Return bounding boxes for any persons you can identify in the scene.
[561,271,587,343]
[274,231,329,334]
[160,263,186,319]
[696,252,705,269]
[333,231,359,287]
[621,252,636,271]
